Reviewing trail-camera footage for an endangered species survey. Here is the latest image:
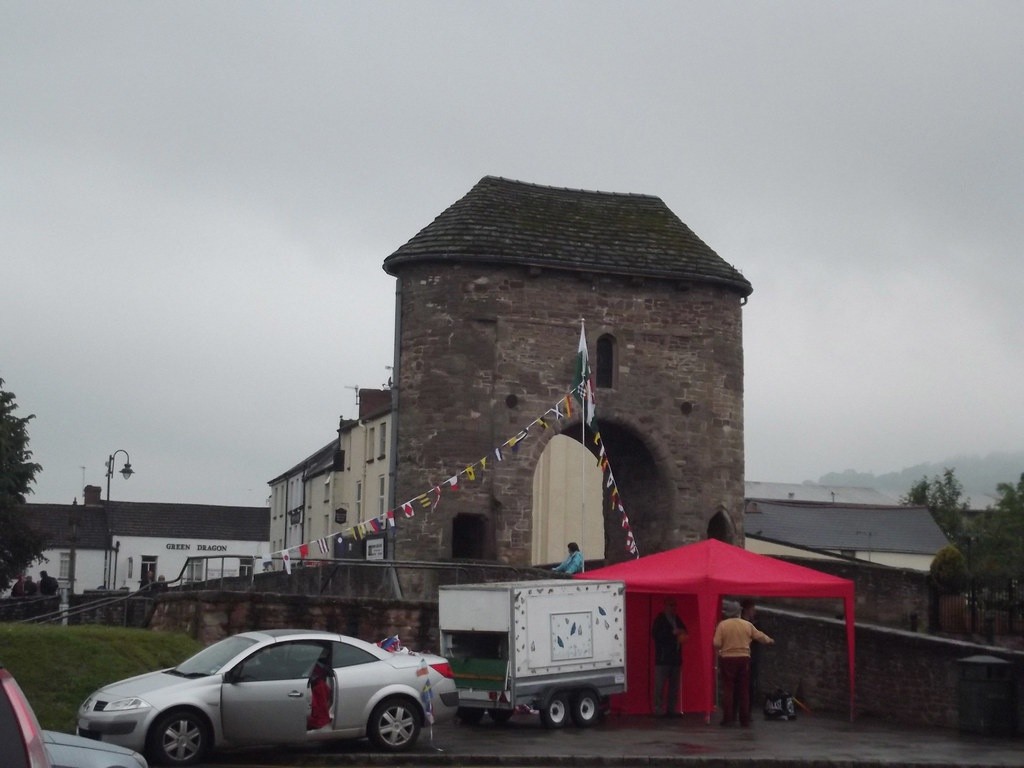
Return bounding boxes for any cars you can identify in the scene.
[38,729,148,768]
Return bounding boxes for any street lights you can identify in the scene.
[101,449,135,594]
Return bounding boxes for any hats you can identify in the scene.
[722,600,743,617]
[736,596,755,608]
[25,576,32,579]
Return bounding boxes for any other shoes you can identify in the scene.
[740,719,749,728]
[666,709,680,719]
[656,710,666,719]
[720,721,733,726]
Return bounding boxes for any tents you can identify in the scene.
[572,537,855,723]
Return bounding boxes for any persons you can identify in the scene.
[139,570,156,592]
[155,575,169,592]
[713,602,775,728]
[21,575,37,596]
[38,570,59,595]
[551,542,582,574]
[650,595,690,718]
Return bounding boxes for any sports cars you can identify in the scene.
[75,628,459,768]
